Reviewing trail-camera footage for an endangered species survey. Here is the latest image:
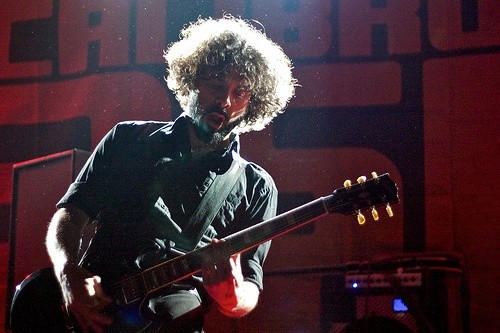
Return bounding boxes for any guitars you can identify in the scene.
[9,171,400,333]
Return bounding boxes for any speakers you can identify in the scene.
[5,147,94,330]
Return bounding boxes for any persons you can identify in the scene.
[46,10,302,333]
